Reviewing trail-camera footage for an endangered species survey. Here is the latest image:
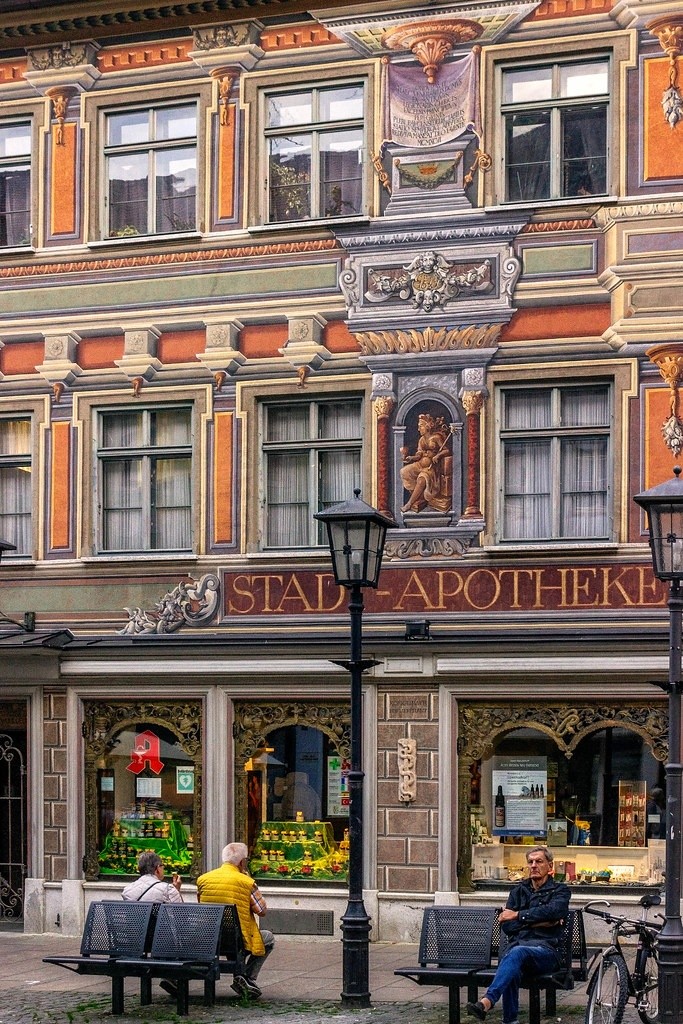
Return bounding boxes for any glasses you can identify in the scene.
[240,857,251,867]
[155,863,166,871]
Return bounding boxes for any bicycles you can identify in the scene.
[584,895,667,1023]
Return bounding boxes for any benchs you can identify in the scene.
[102,899,251,1003]
[427,908,586,1017]
[42,902,226,1014]
[394,906,575,1024]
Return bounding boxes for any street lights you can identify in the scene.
[634,464,682,1024]
[312,489,397,1007]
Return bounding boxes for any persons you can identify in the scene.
[196,842,275,998]
[466,846,572,1024]
[249,774,262,839]
[122,850,186,997]
[646,788,666,841]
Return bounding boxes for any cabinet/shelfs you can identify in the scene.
[98,818,184,869]
[250,820,332,875]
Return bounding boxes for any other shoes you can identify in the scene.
[467,1002,487,1021]
[234,973,262,998]
[158,978,178,998]
[230,982,258,1000]
